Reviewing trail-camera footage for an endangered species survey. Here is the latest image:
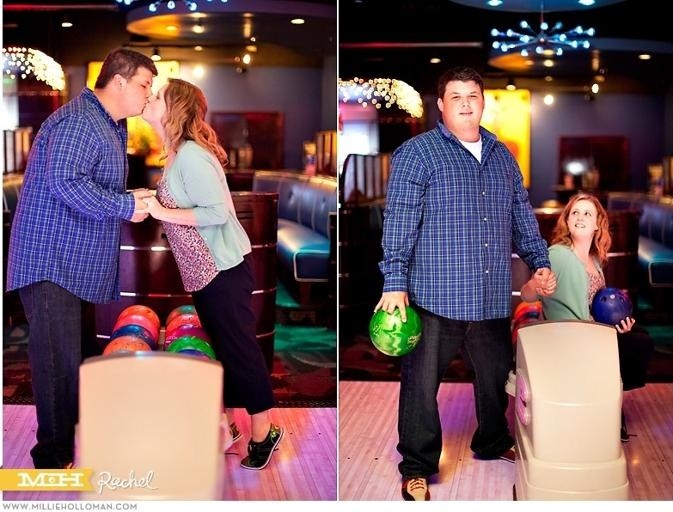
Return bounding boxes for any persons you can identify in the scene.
[373,67,557,500]
[7,49,158,468]
[521,194,635,442]
[133,79,284,470]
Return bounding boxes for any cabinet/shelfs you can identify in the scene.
[557,137,631,210]
[210,111,285,170]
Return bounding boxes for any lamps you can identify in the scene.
[3,47,65,91]
[490,1,595,56]
[339,77,423,119]
[150,47,161,61]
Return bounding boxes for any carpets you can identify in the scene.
[340,381,673,501]
[3,405,336,500]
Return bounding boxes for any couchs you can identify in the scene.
[608,192,673,326]
[252,170,337,327]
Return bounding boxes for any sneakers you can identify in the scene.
[402,475,430,501]
[241,423,283,470]
